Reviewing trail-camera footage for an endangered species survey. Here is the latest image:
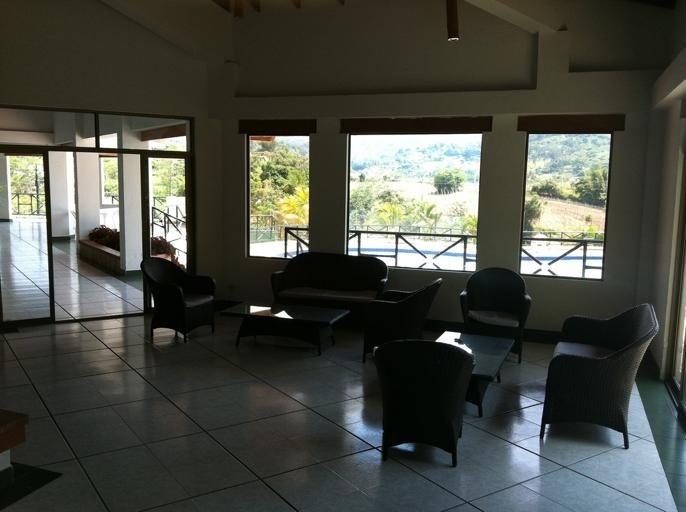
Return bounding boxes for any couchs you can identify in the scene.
[269,250,391,321]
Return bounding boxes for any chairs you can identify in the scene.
[360,274,445,362]
[374,336,476,468]
[459,266,534,364]
[538,300,659,450]
[139,256,218,344]
